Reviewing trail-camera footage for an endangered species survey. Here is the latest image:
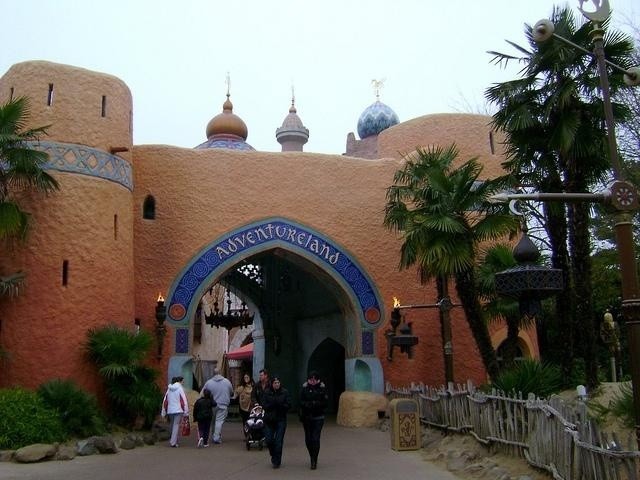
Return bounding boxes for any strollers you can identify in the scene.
[243,402,267,451]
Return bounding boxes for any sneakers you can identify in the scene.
[197,437,210,447]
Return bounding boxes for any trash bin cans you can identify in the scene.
[227,367,247,396]
[390,398,421,451]
[198,360,216,389]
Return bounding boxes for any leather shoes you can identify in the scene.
[311,460,317,469]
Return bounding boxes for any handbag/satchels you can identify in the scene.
[182,421,190,436]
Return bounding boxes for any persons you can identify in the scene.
[161,367,329,470]
